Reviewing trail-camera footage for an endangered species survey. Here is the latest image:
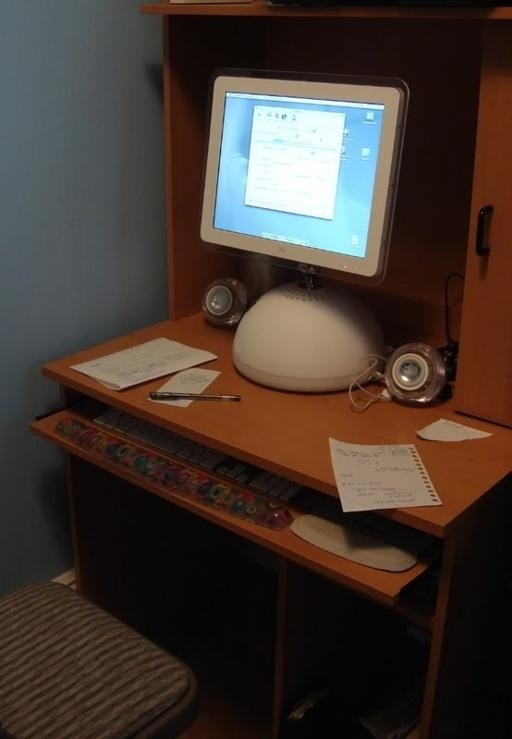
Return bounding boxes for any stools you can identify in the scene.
[0,580,202,739]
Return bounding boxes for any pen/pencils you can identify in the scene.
[149,392,241,401]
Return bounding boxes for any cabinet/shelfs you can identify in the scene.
[137,0,512,428]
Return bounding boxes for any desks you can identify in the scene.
[23,313,512,739]
